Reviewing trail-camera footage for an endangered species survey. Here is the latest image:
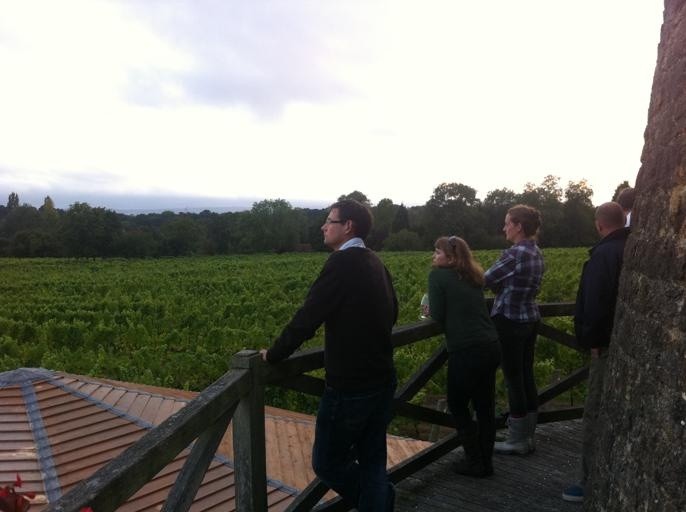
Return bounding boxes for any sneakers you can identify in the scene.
[562,486,586,502]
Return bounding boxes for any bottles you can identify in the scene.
[419,292,431,320]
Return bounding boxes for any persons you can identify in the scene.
[484,204,544,456]
[423,236,504,483]
[563,202,631,501]
[616,187,634,228]
[260,199,400,511]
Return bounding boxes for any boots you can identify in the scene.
[493,411,538,455]
